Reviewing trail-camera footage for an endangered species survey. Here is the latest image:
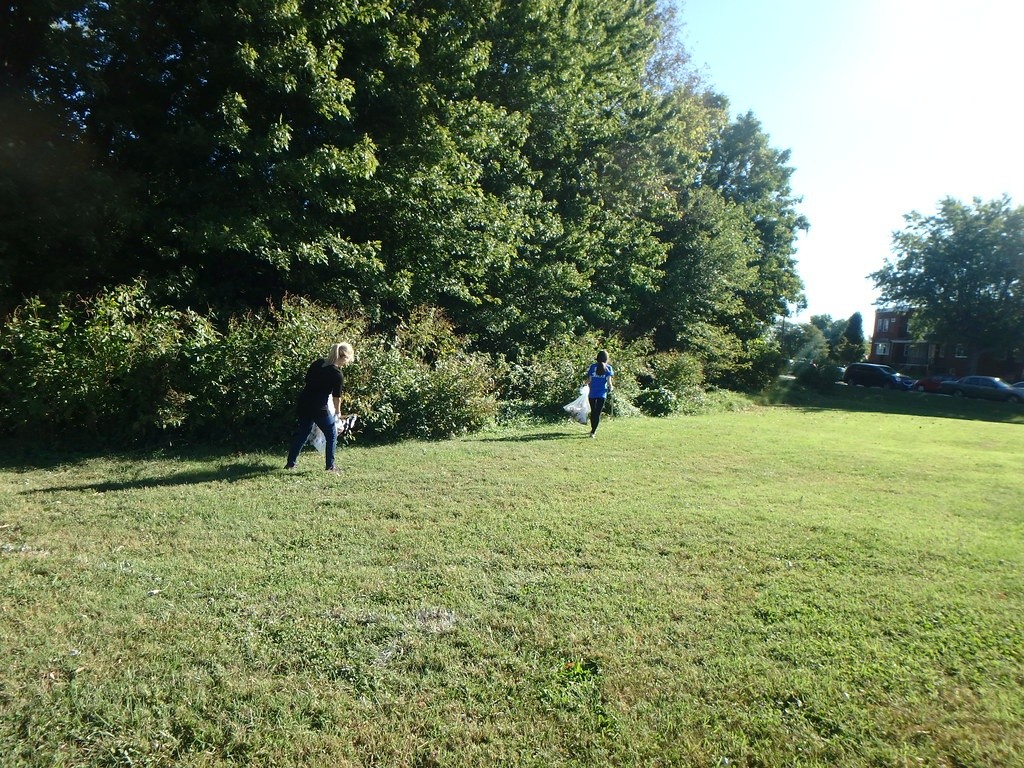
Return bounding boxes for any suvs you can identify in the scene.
[843,362,914,392]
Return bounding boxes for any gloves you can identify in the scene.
[583,384,587,387]
[608,387,613,393]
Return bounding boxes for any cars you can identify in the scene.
[912,375,1024,405]
[788,360,847,382]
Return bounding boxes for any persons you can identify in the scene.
[284,343,354,471]
[583,350,616,438]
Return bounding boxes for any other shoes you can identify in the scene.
[284,464,296,469]
[328,466,339,472]
[590,432,596,438]
